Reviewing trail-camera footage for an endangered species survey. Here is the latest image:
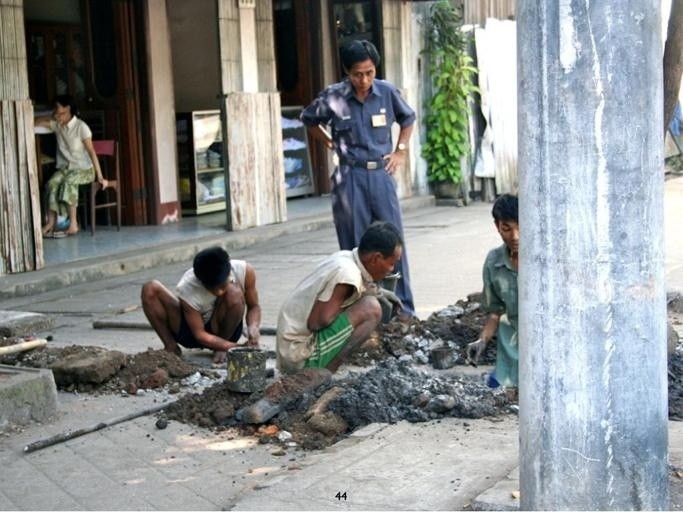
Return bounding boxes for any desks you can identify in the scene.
[86,105,121,141]
[35,116,53,191]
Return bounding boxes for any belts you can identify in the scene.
[340,158,388,170]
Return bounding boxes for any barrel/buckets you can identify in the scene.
[226,347,267,392]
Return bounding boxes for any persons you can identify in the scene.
[34,94,109,237]
[276,220,406,377]
[299,40,415,322]
[465,193,518,389]
[140,246,262,363]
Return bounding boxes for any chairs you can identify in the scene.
[79,140,121,235]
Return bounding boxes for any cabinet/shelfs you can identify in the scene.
[25,20,91,109]
[176,105,315,216]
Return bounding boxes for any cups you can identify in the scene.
[431,346,454,369]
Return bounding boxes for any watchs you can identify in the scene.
[396,143,408,151]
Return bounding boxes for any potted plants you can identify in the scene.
[419,0,482,199]
